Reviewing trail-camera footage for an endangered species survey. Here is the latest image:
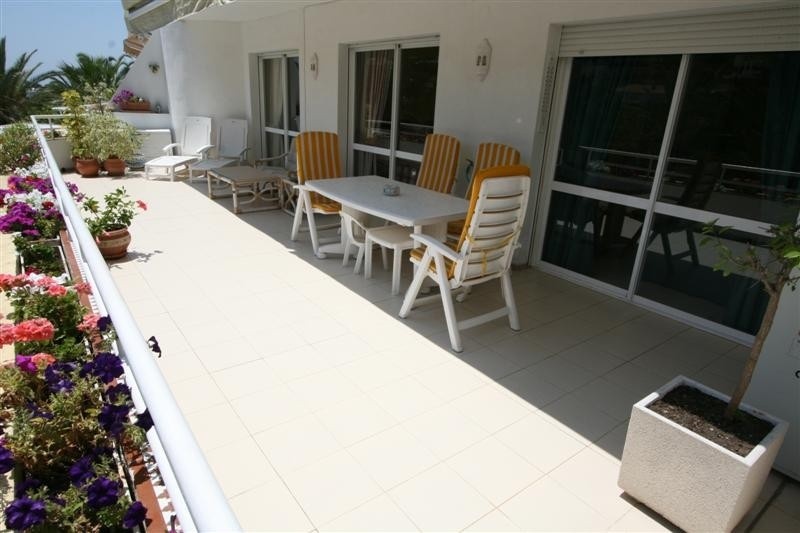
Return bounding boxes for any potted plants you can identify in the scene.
[60,85,99,176]
[618,196,800,533]
[81,108,147,176]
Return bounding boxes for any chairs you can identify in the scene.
[339,210,389,275]
[291,132,380,262]
[447,142,522,241]
[543,139,724,279]
[397,165,533,353]
[188,118,248,190]
[143,117,217,182]
[254,138,297,203]
[363,132,463,296]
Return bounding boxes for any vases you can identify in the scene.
[20,224,62,247]
[119,101,151,112]
[93,223,132,262]
[20,245,73,285]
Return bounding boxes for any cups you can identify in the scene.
[384,182,401,196]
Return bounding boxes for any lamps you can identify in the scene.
[474,38,492,83]
[309,53,318,81]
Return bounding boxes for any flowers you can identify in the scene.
[82,186,148,238]
[0,137,148,533]
[112,88,146,103]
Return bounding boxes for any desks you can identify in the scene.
[579,170,684,279]
[303,173,472,298]
[206,165,284,215]
